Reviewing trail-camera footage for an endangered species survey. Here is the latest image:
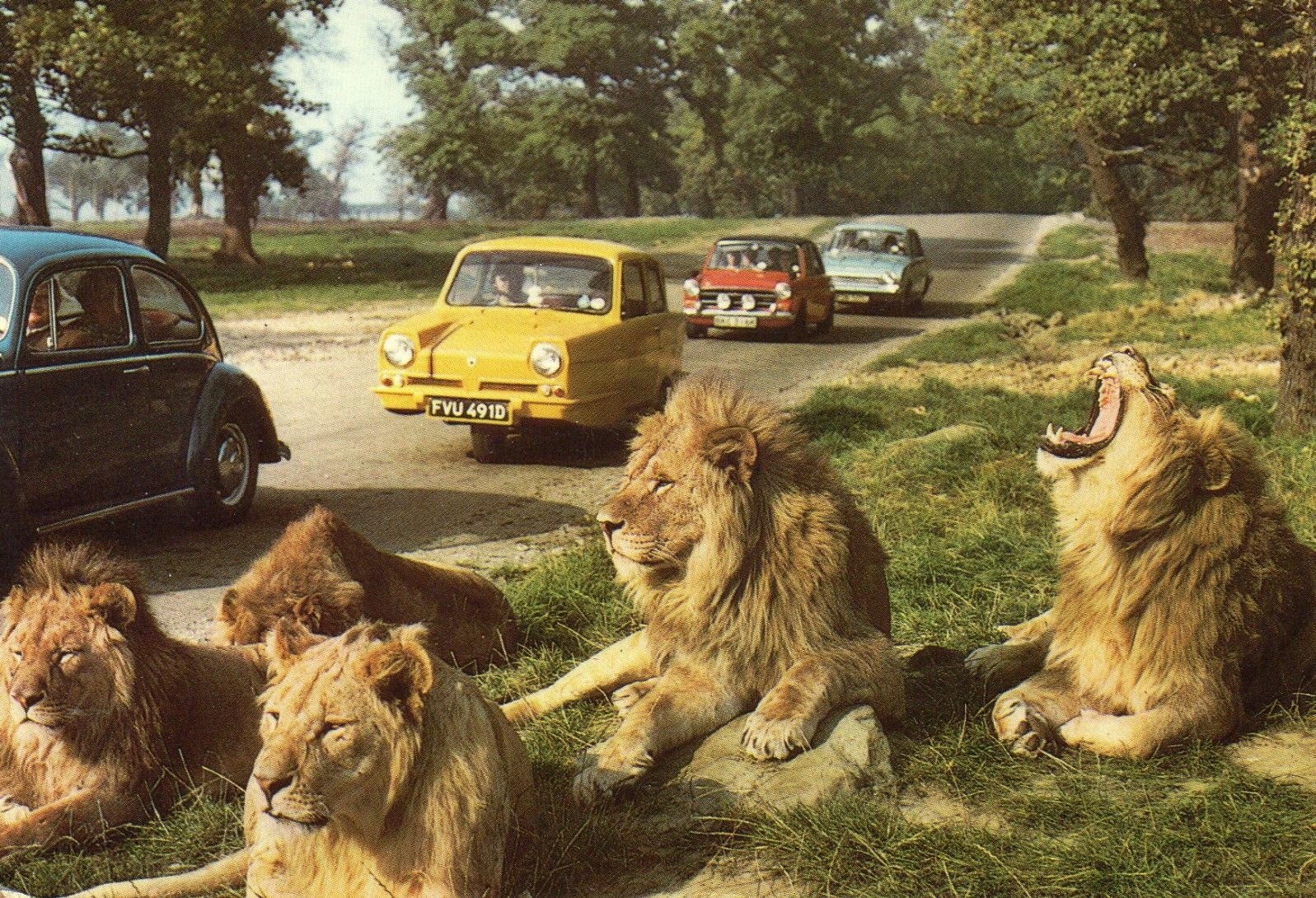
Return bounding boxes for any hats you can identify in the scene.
[490,261,524,283]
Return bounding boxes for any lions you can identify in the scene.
[0,506,540,898]
[967,344,1316,794]
[498,377,914,813]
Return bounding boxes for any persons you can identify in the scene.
[0,268,155,352]
[482,260,529,306]
[840,230,907,256]
[726,247,799,276]
[588,271,628,311]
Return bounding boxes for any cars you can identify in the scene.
[683,234,836,341]
[0,225,292,593]
[820,223,935,314]
[374,236,688,465]
